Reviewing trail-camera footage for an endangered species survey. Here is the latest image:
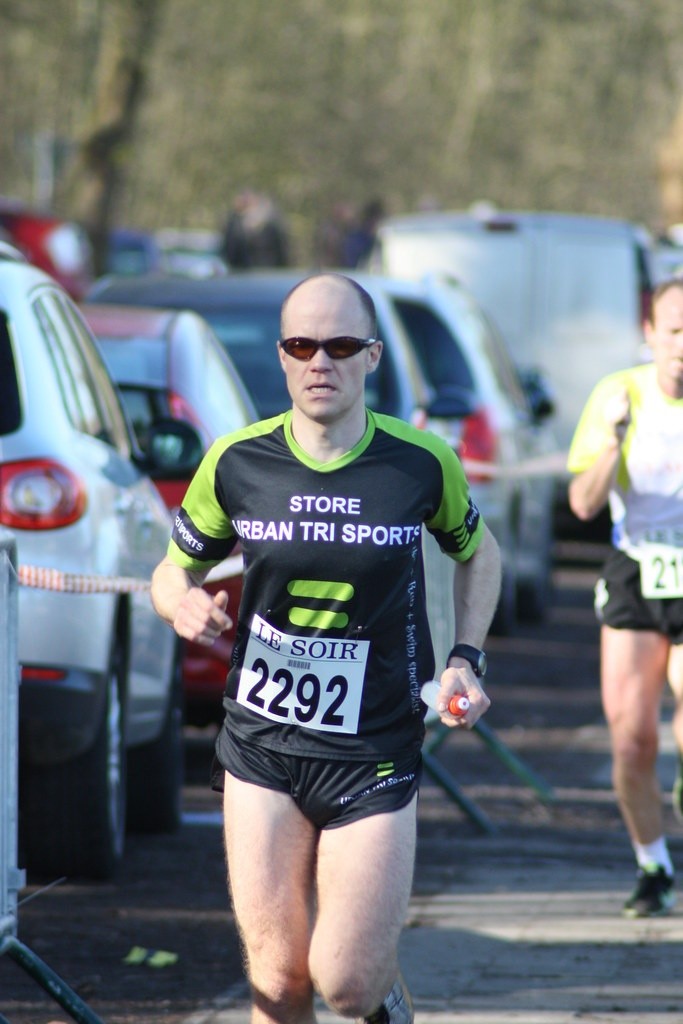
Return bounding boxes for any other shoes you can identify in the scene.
[363,971,414,1024]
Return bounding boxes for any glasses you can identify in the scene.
[279,336,378,362]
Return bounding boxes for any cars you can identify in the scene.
[2,204,229,296]
[74,299,271,713]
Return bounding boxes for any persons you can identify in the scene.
[220,194,385,271]
[151,275,502,1024]
[567,279,683,915]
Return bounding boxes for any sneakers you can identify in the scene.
[622,862,679,918]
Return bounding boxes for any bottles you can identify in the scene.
[421,681,469,719]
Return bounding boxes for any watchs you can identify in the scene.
[446,643,488,678]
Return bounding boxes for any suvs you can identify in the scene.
[2,240,194,873]
[367,274,567,633]
[78,270,477,725]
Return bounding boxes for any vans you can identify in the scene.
[372,201,668,534]
